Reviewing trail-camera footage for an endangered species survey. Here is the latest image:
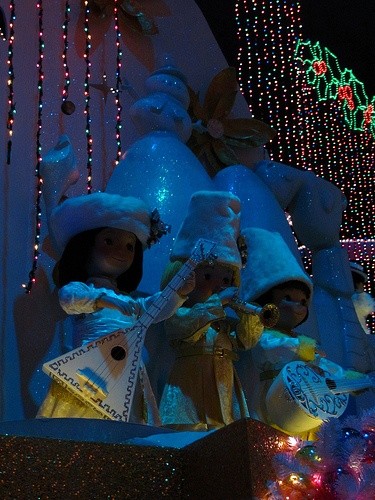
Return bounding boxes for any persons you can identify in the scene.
[156,257,263,430]
[237,280,327,430]
[36,226,197,424]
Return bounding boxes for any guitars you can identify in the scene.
[261,359,375,433]
[36,237,217,424]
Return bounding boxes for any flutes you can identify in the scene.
[219,286,278,328]
